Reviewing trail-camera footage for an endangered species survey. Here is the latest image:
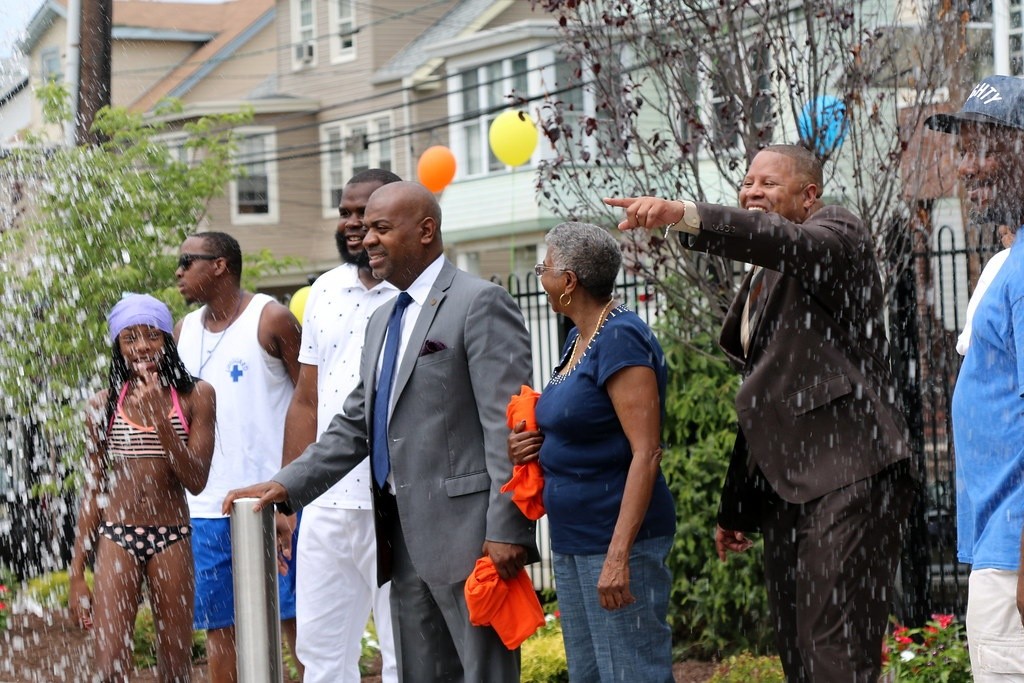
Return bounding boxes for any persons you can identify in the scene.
[284,168,402,682]
[170,231,303,683]
[799,94,848,158]
[604,144,909,683]
[506,220,678,683]
[224,180,542,682]
[925,76,1024,683]
[67,290,216,683]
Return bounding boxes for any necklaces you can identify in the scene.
[198,292,244,377]
[566,297,614,376]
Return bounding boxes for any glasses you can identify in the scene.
[534,263,572,276]
[177,253,219,270]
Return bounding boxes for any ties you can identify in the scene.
[371,292,414,491]
[748,266,764,344]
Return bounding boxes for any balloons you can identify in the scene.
[419,146,456,192]
[490,111,538,166]
[289,287,314,326]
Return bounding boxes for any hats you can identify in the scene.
[108,292,174,344]
[923,75,1023,134]
